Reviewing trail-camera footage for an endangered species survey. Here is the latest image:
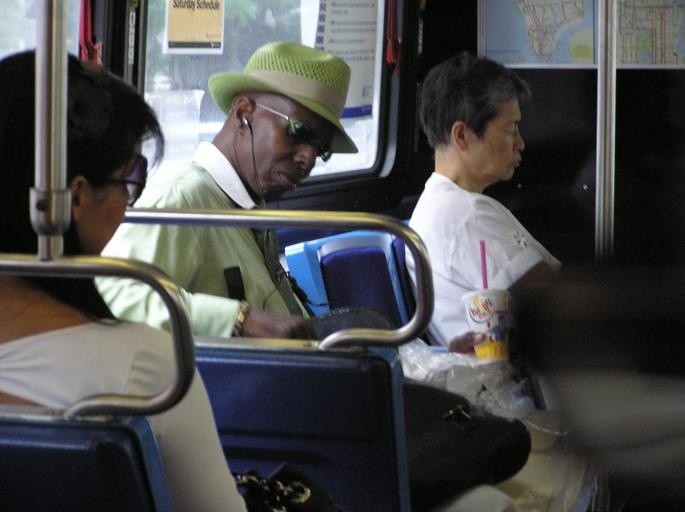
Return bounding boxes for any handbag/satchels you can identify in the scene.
[304,302,532,512]
[232,458,344,512]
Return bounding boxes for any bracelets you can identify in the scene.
[231,298,252,339]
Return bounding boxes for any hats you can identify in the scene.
[208,41,358,154]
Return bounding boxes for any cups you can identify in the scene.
[461,290,512,366]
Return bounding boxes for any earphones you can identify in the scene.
[239,114,250,133]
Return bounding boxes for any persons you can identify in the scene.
[0,44,258,512]
[95,39,613,512]
[402,52,685,489]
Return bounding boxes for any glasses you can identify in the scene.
[256,101,333,162]
[107,153,149,208]
